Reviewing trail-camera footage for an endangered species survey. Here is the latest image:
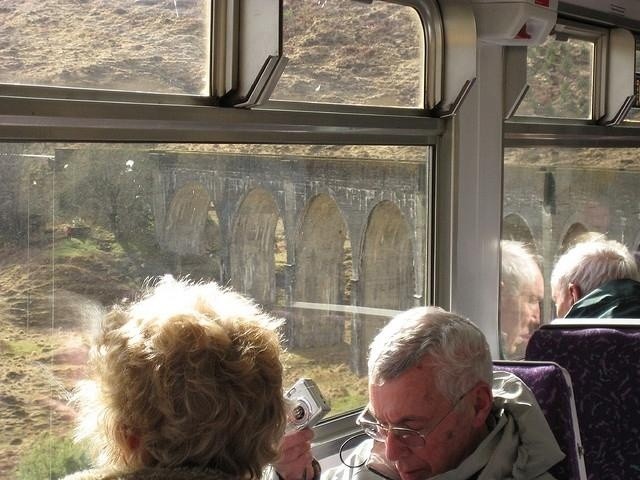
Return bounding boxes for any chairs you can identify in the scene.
[491,318,639,479]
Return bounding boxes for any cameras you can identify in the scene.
[283,379,332,435]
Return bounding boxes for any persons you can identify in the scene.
[546,235,640,317]
[500,239,544,360]
[62,279,283,480]
[266,302,565,480]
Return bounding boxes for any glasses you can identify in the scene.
[354,392,469,449]
